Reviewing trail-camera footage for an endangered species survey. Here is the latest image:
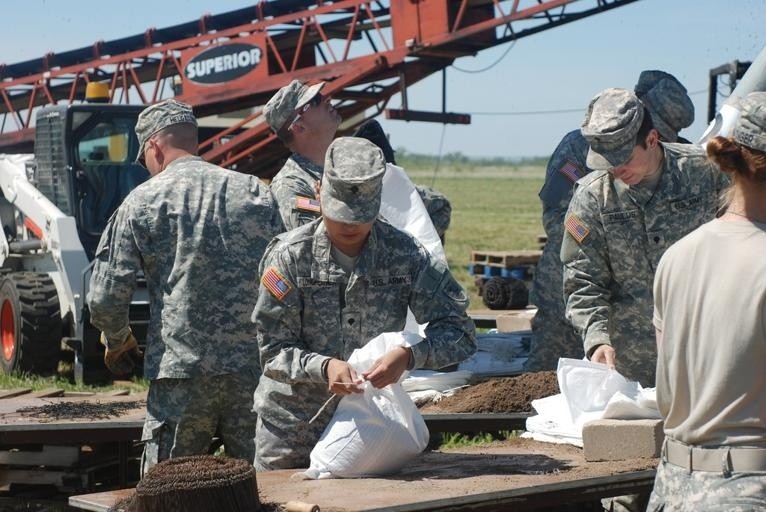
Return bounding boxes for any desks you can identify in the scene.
[418,379,535,434]
[0,384,150,499]
[68,443,660,512]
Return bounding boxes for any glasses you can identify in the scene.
[137,144,151,170]
[286,91,322,131]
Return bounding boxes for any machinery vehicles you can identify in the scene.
[0,0,639,391]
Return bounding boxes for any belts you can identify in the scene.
[664,439,766,473]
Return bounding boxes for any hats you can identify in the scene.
[730,90,766,155]
[579,87,645,172]
[261,79,326,134]
[632,68,695,144]
[319,135,386,227]
[132,97,198,166]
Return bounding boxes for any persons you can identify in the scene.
[81,96,287,485]
[559,89,734,397]
[263,76,453,242]
[642,92,766,511]
[244,134,479,476]
[524,68,699,374]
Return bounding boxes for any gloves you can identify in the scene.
[104,334,144,376]
